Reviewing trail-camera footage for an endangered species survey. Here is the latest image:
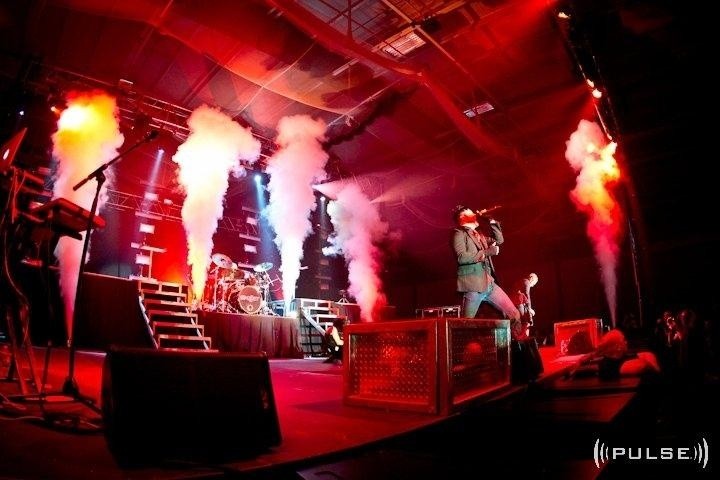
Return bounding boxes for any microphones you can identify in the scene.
[476,206,500,217]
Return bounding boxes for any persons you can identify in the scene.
[452,203,523,325]
[652,311,673,338]
[502,271,539,338]
[667,308,697,349]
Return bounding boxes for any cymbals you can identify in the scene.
[237,262,254,268]
[212,253,232,269]
[254,262,273,272]
[222,267,244,279]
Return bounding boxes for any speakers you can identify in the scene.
[98,346,282,475]
[511,337,543,386]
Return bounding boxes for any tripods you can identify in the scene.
[0,130,159,416]
[204,271,239,314]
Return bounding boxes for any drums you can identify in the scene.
[228,286,261,314]
